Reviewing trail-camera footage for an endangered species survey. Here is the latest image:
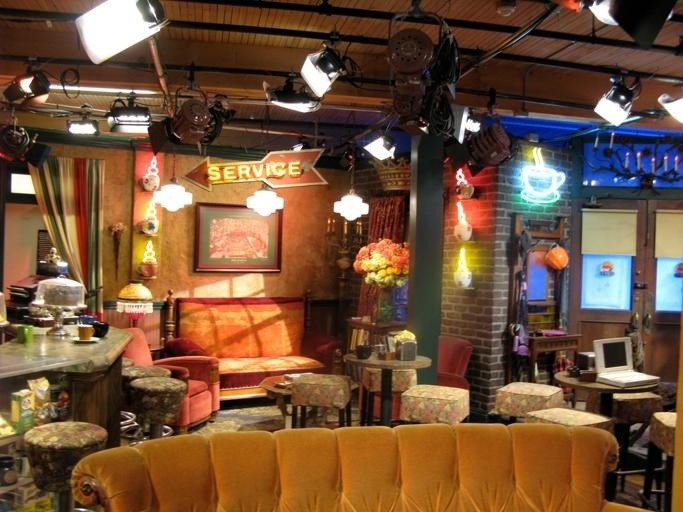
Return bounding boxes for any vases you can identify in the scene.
[378,288,392,323]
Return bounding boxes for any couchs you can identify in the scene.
[69,420,650,511]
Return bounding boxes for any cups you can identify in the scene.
[79,315,98,325]
[78,325,95,340]
[89,320,110,338]
[452,183,475,288]
[374,344,386,360]
[356,344,372,358]
[139,177,160,192]
[16,324,33,344]
[138,220,160,235]
[136,262,159,277]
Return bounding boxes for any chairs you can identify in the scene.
[117,326,221,433]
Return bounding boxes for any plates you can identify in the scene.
[71,336,101,342]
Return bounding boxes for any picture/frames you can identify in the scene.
[190,200,284,275]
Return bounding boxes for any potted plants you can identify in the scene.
[394,330,418,361]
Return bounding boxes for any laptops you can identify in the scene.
[593,337,660,388]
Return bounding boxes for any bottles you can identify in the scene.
[0,454,18,487]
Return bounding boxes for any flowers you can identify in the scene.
[352,237,410,288]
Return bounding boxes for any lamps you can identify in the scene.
[245,187,288,216]
[115,278,153,326]
[590,1,683,128]
[264,42,345,114]
[13,1,195,227]
[331,104,483,238]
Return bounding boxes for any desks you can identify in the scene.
[552,370,659,415]
[259,374,357,427]
[342,350,431,427]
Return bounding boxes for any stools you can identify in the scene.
[131,377,189,440]
[119,355,138,436]
[23,418,107,511]
[523,406,614,435]
[355,368,417,422]
[611,393,664,491]
[397,385,471,426]
[636,411,677,510]
[121,366,175,442]
[289,373,351,428]
[493,379,563,421]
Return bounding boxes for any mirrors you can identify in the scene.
[525,246,553,306]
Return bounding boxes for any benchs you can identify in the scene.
[162,290,345,407]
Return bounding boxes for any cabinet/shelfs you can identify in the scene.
[453,238,474,291]
[0,410,56,511]
[515,333,582,384]
[345,314,406,391]
